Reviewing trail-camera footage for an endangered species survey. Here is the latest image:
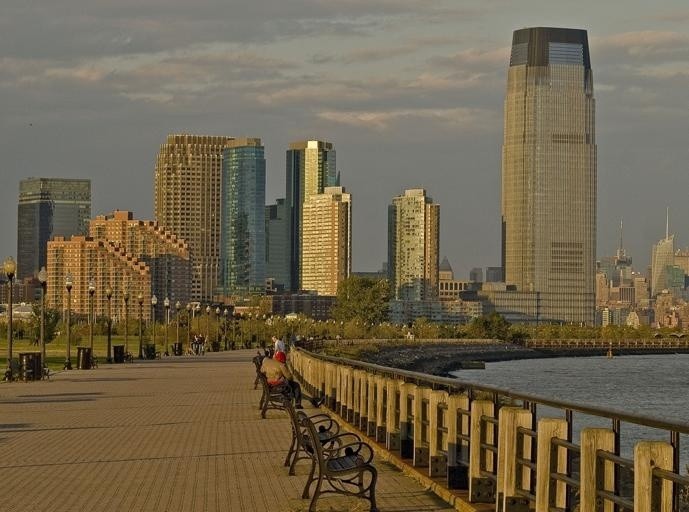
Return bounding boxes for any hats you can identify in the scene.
[275,351,287,362]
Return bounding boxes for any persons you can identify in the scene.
[192,333,199,357]
[196,333,207,356]
[259,332,345,409]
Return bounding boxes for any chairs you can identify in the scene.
[252,355,378,512]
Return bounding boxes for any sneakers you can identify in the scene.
[311,398,324,407]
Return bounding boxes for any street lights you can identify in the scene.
[163,296,171,356]
[86,278,96,366]
[137,291,144,358]
[1,255,17,384]
[150,293,159,359]
[175,298,458,364]
[36,266,49,378]
[103,283,115,362]
[121,290,132,359]
[63,272,74,371]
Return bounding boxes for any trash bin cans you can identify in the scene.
[229,341,235,349]
[77,347,91,369]
[20,352,41,381]
[148,344,155,359]
[213,341,220,352]
[261,340,265,348]
[175,343,182,356]
[246,340,251,349]
[114,346,124,363]
